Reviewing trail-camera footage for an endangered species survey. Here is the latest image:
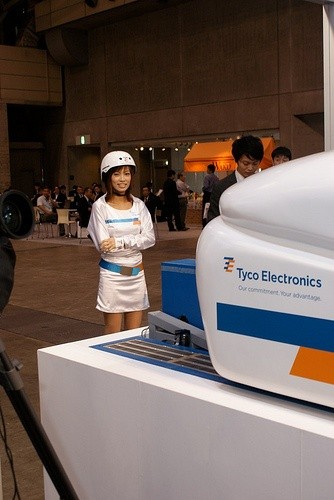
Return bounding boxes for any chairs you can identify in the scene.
[34,207,54,239]
[56,208,76,239]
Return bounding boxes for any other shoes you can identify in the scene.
[169,227,190,231]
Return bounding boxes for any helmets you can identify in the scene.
[100,151,136,181]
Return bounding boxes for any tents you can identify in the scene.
[182,137,278,172]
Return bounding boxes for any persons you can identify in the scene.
[87,150,154,334]
[0,181,104,316]
[143,135,290,232]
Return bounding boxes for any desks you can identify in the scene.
[36,326,334,500]
